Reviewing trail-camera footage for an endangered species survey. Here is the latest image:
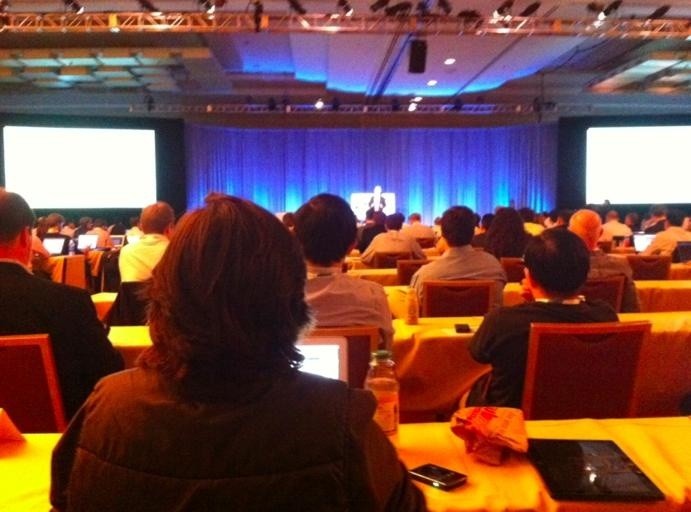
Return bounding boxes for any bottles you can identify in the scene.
[68,239,76,255]
[364,352,402,437]
[402,287,421,326]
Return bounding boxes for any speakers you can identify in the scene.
[407,39,428,72]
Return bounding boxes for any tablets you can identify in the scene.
[524,438,666,502]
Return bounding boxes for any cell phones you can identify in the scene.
[455,323,470,333]
[407,462,468,489]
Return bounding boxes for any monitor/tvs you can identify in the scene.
[42,237,67,256]
[110,234,126,247]
[76,233,98,251]
[291,335,349,384]
[632,233,656,254]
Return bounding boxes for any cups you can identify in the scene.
[624,239,632,248]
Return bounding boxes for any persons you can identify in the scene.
[1,182,691,512]
